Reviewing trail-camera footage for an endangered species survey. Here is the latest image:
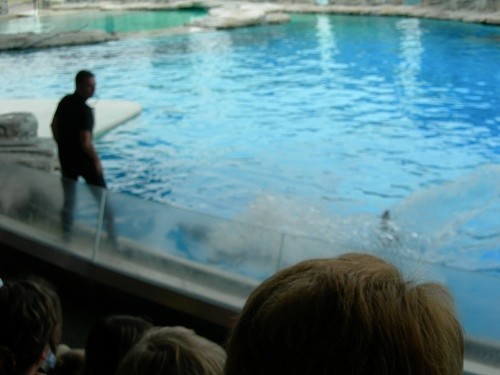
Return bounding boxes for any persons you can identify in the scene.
[117,324,229,375]
[37,341,87,375]
[220,253,463,375]
[1,277,64,375]
[52,69,133,255]
[85,316,157,375]
